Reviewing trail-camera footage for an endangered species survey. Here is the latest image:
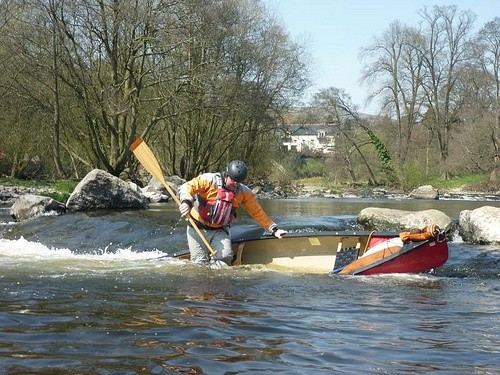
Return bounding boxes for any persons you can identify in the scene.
[179,160,288,266]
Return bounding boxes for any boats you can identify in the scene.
[159,230,449,275]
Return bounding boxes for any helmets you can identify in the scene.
[224,160,248,182]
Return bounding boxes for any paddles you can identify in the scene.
[130,137,217,258]
